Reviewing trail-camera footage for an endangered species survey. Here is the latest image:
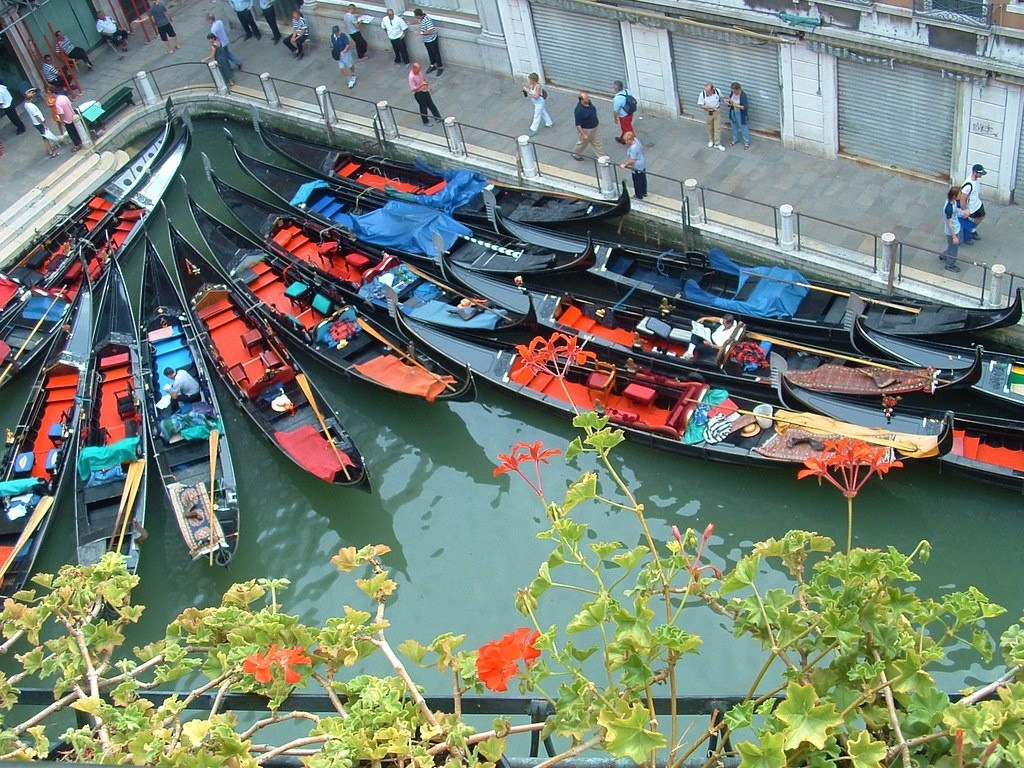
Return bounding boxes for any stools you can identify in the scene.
[259,350,282,379]
[9,250,82,288]
[345,253,370,276]
[15,452,36,475]
[283,282,332,319]
[622,383,656,414]
[228,363,249,385]
[46,449,62,476]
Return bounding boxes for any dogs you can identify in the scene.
[52,130,70,148]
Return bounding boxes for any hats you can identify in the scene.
[331,26,340,33]
[972,164,988,176]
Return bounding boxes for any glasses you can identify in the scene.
[45,59,52,62]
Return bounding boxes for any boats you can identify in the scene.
[178,172,478,405]
[136,205,241,569]
[74,227,149,618]
[434,248,985,401]
[218,124,598,286]
[0,95,194,393]
[774,370,1023,489]
[490,205,1023,343]
[385,283,954,474]
[158,196,374,496]
[197,151,539,350]
[0,243,96,615]
[848,313,1024,420]
[244,98,632,232]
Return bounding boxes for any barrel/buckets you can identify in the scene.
[754,404,773,429]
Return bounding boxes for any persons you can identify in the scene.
[613,80,635,145]
[148,0,180,54]
[0,31,96,158]
[697,83,729,151]
[329,26,356,88]
[344,4,369,61]
[202,0,309,88]
[961,164,987,244]
[571,92,606,161]
[524,73,553,137]
[728,82,750,150]
[96,11,129,52]
[163,367,201,414]
[381,9,411,68]
[620,131,647,199]
[939,187,970,273]
[683,314,737,357]
[408,63,444,126]
[406,9,443,76]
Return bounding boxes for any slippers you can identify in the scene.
[164,50,174,55]
[173,45,181,49]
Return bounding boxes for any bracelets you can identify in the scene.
[421,31,424,34]
[343,49,346,53]
[529,92,532,95]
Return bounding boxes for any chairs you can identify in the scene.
[67,58,83,72]
[105,38,119,52]
[48,410,68,449]
[240,316,266,357]
[303,34,311,55]
[318,229,345,268]
[114,381,136,421]
[585,359,618,410]
[682,252,712,292]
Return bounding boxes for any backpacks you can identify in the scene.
[617,90,637,114]
[535,84,548,99]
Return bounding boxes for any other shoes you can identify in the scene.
[422,121,433,127]
[614,137,625,145]
[121,39,130,53]
[528,130,538,137]
[633,193,648,199]
[88,65,95,71]
[436,118,444,123]
[348,75,357,88]
[16,127,26,136]
[359,56,443,78]
[707,140,749,152]
[570,154,584,161]
[238,63,242,70]
[938,231,982,273]
[542,124,553,128]
[243,34,306,61]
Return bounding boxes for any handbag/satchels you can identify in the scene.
[331,48,340,61]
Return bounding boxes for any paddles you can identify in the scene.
[741,269,921,313]
[685,397,919,451]
[0,495,54,580]
[486,179,618,207]
[295,374,351,481]
[406,265,513,322]
[209,429,219,565]
[0,284,68,382]
[746,331,952,385]
[108,459,146,553]
[355,317,456,391]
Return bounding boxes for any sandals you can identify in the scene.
[47,144,81,158]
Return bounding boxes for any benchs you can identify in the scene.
[84,86,135,130]
[309,304,358,346]
[637,319,743,364]
[247,364,295,400]
[359,255,400,286]
